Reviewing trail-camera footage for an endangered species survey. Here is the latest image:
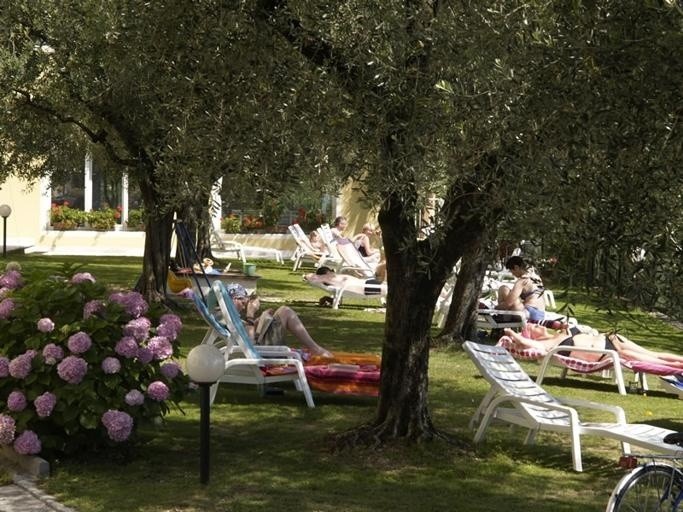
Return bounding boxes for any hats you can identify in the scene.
[226,284,247,299]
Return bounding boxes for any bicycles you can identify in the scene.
[604,453,683,511]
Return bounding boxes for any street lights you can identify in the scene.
[0,204,13,260]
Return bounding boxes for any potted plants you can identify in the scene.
[128,208,146,231]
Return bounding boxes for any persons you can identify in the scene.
[490,256,545,343]
[228,288,335,373]
[309,216,381,278]
[304,266,388,297]
[504,322,683,369]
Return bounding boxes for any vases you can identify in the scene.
[108,221,116,230]
[53,222,64,229]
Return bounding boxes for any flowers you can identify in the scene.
[50,200,73,220]
[102,202,123,220]
